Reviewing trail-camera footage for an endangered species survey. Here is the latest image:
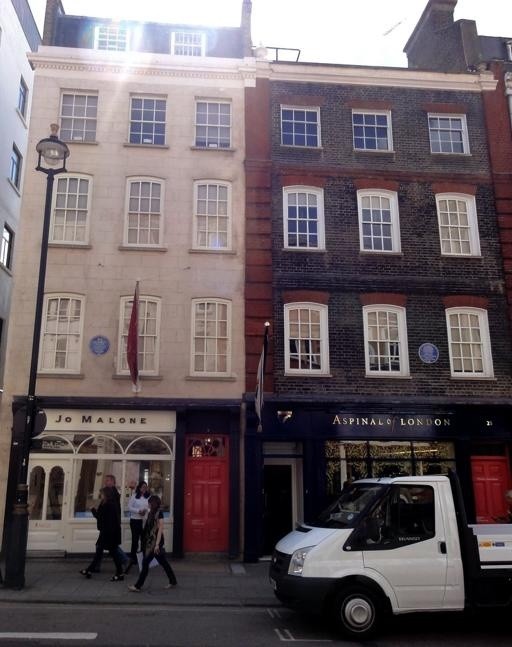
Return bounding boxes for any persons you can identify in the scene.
[81,473,177,593]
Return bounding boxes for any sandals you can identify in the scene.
[79,568,93,579]
[113,574,125,581]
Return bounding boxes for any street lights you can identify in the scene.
[5,122,71,590]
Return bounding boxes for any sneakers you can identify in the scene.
[127,584,141,592]
[164,583,177,589]
[124,558,134,573]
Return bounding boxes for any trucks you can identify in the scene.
[266,475,512,636]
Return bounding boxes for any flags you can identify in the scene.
[254,344,264,423]
[126,291,138,387]
